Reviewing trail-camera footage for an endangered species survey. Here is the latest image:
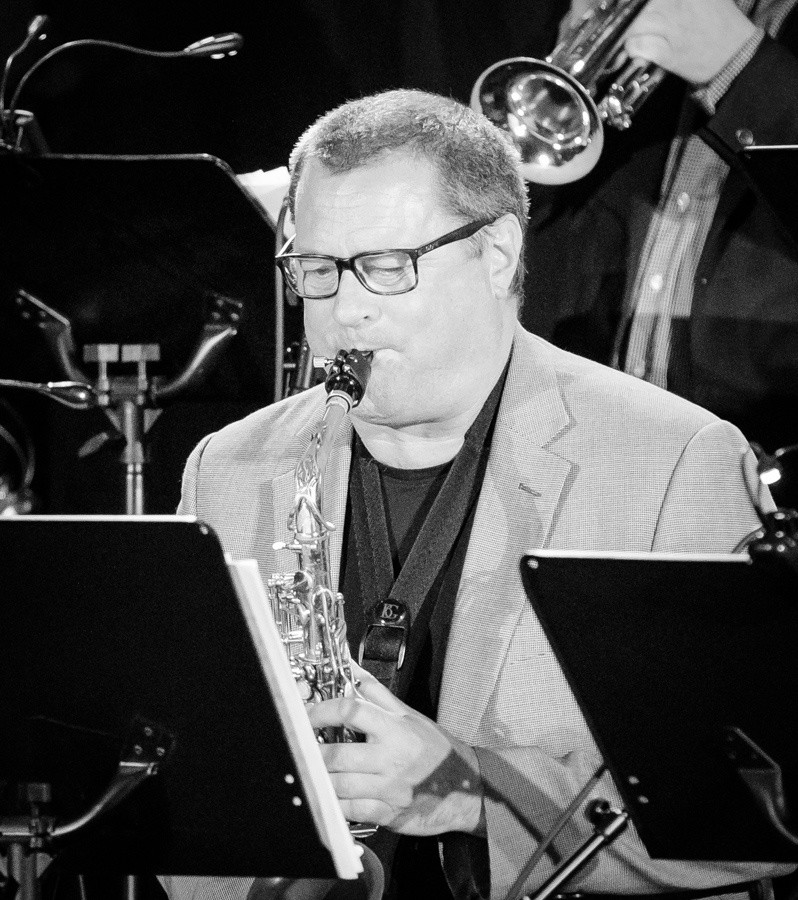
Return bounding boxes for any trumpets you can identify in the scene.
[469,0,666,185]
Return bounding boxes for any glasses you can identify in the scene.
[273,210,510,299]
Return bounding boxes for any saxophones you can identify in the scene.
[246,348,385,900]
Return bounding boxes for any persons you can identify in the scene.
[514,0,798,519]
[154,92,798,900]
